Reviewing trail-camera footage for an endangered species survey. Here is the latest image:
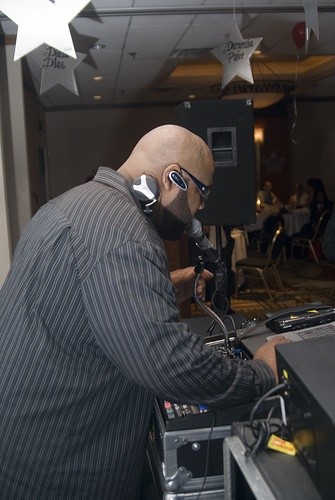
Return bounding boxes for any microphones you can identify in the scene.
[184,218,222,269]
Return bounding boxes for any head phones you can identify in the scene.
[131,172,160,217]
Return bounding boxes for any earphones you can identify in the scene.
[169,170,188,192]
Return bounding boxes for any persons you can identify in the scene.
[0,124,289,500]
[301,177,329,237]
[256,179,277,209]
[290,181,308,207]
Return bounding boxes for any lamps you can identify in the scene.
[209,48,296,110]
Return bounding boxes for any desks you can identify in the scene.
[251,208,312,254]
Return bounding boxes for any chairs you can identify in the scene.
[289,209,330,266]
[232,224,287,300]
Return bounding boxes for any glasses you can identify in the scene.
[180,167,213,203]
